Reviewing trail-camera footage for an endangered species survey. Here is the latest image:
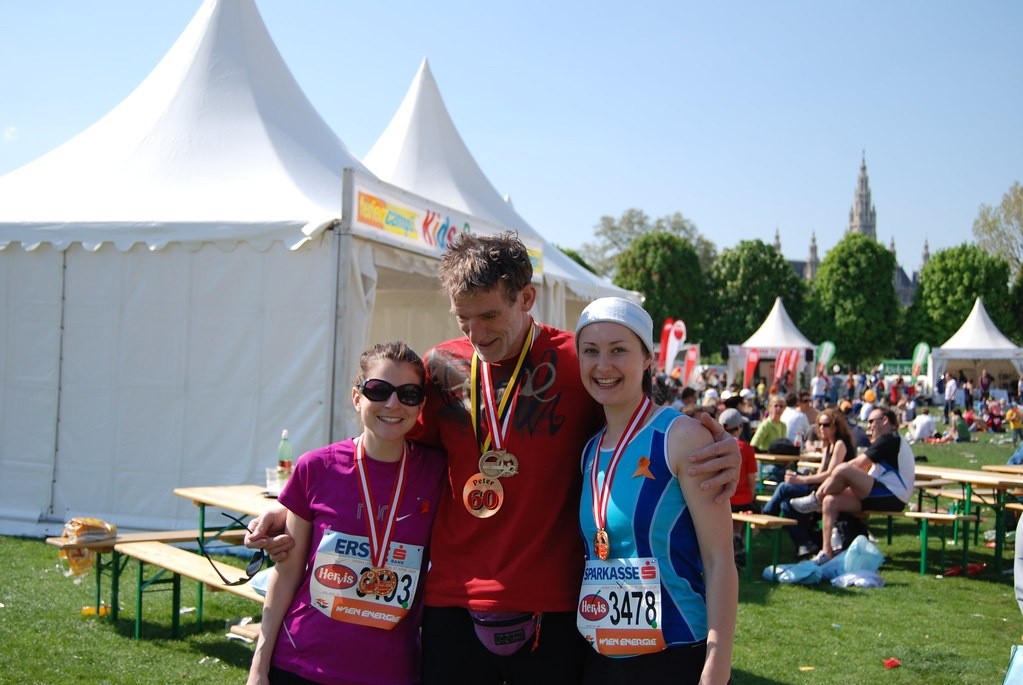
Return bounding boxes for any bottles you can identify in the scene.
[277,429,292,493]
[831,527,842,551]
[794,432,802,453]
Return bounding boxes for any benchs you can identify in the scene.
[46,529,248,624]
[113,541,267,643]
[722,479,1023,584]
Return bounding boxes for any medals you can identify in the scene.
[360,568,396,596]
[478,451,518,479]
[594,530,609,560]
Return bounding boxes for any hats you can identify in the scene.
[704,388,718,398]
[1010,401,1017,407]
[739,388,755,399]
[718,408,749,430]
[864,390,876,403]
[721,390,738,400]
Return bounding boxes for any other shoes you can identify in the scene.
[798,544,810,556]
[790,493,822,513]
[811,550,829,565]
[732,535,747,566]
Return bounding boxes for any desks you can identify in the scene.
[916,466,1023,576]
[174,485,285,632]
[753,453,822,468]
[951,389,1009,405]
[981,465,1023,476]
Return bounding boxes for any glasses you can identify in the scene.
[867,417,884,425]
[818,423,832,428]
[357,378,426,406]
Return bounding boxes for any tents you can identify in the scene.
[741,296,817,391]
[0,0,647,558]
[926,296,1023,403]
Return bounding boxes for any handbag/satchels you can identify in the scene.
[467,610,541,655]
[762,534,884,588]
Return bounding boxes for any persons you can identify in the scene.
[243,233,742,685]
[650,369,1023,569]
[574,296,739,685]
[245,342,446,685]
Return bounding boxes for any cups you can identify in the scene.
[856,446,868,458]
[266,466,295,496]
[814,440,824,454]
